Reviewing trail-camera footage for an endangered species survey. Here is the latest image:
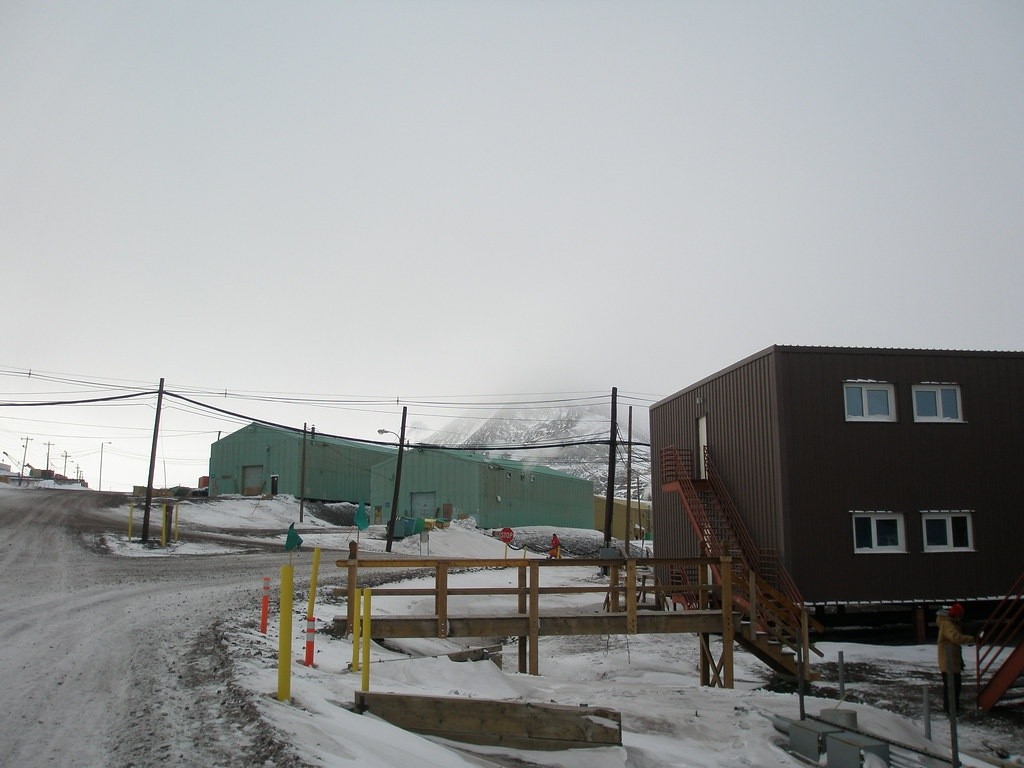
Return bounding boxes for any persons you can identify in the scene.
[546,533,562,561]
[935,603,984,718]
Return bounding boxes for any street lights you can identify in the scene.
[377,406,409,553]
[98,440,113,490]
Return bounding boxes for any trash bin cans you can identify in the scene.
[388,515,425,538]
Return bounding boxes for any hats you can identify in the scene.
[949,603,963,619]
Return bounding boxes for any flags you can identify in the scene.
[285,524,303,558]
[353,498,369,531]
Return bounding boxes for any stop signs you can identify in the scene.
[499,528,514,543]
